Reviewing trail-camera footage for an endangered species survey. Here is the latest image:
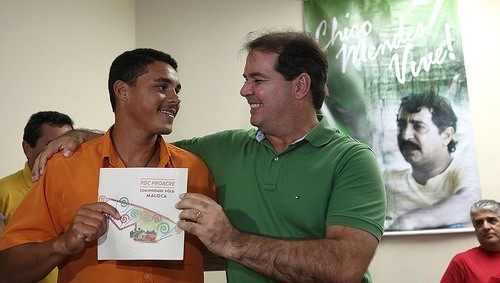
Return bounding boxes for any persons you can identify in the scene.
[0,112,75,283]
[440,200,500,283]
[0,48,216,283]
[382,91,481,230]
[32,33,387,283]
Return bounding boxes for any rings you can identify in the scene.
[196,211,201,218]
